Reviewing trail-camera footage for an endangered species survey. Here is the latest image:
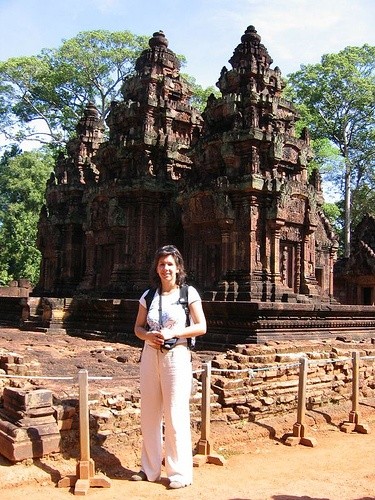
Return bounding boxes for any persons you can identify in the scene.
[130,244,207,489]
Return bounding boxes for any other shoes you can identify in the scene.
[169,481,185,489]
[131,471,147,481]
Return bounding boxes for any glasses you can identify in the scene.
[155,247,177,256]
